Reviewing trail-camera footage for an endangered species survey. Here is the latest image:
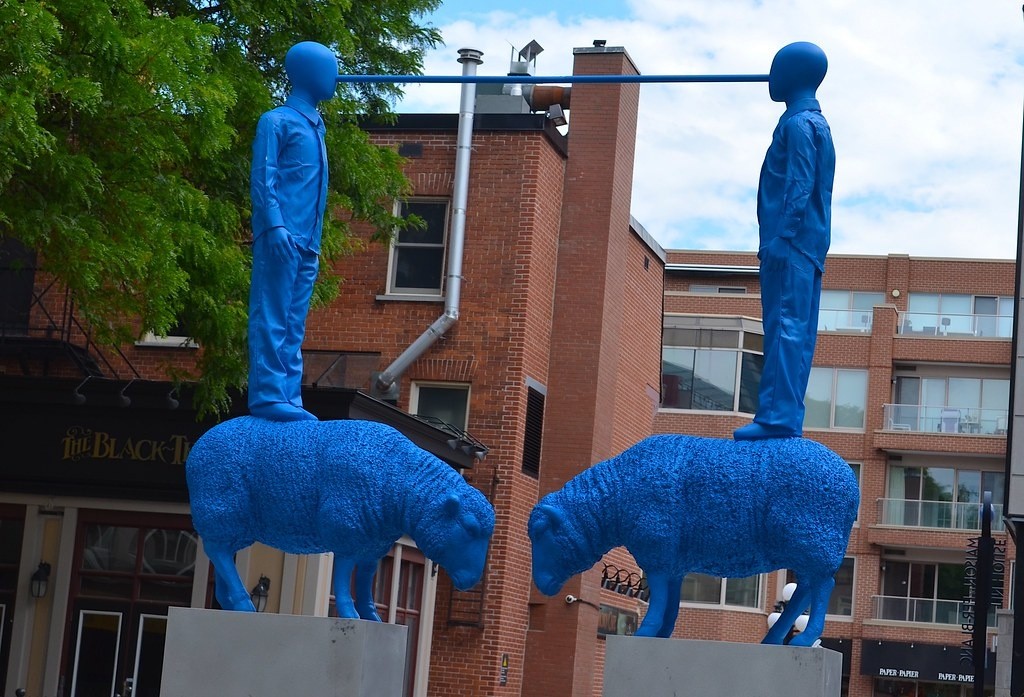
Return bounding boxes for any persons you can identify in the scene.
[732,40,835,441]
[247,42,337,423]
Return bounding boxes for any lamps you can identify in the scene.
[249,572,275,612]
[607,569,635,598]
[601,562,611,589]
[119,375,138,409]
[31,558,53,598]
[620,572,646,600]
[165,379,184,410]
[70,373,92,407]
[601,564,625,594]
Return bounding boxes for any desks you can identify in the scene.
[959,422,979,433]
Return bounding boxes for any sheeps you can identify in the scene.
[527,432,863,647]
[182,411,498,623]
